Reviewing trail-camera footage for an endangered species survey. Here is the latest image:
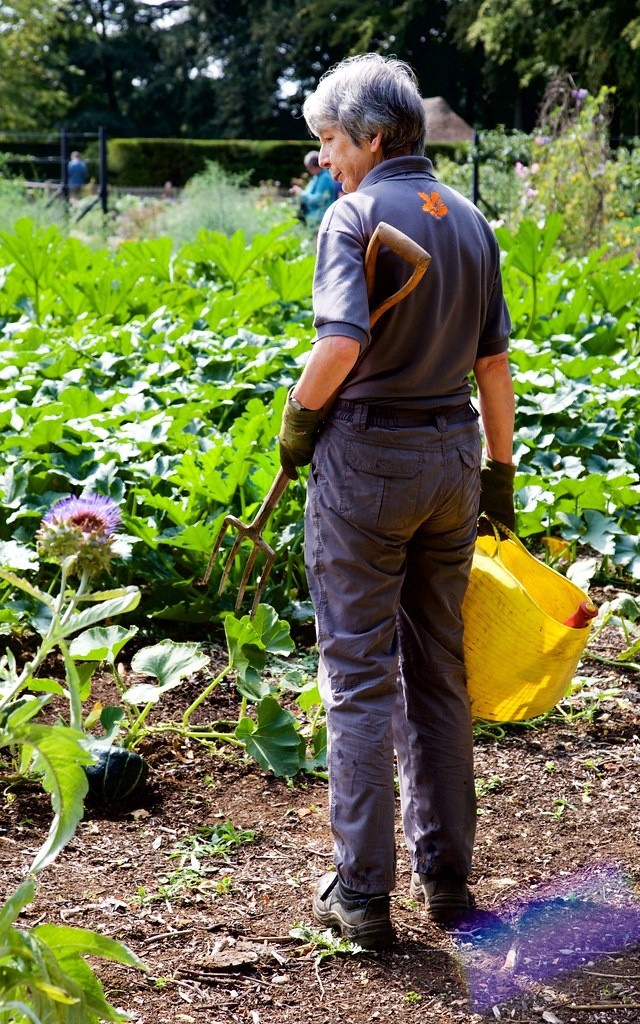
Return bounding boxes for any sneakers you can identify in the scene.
[411,870,470,915]
[313,871,391,948]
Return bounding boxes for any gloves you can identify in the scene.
[476,457,518,541]
[279,384,324,479]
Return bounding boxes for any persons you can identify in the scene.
[288,150,347,260]
[67,151,87,196]
[279,59,517,947]
[160,182,175,205]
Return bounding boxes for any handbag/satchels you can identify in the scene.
[462,511,597,725]
